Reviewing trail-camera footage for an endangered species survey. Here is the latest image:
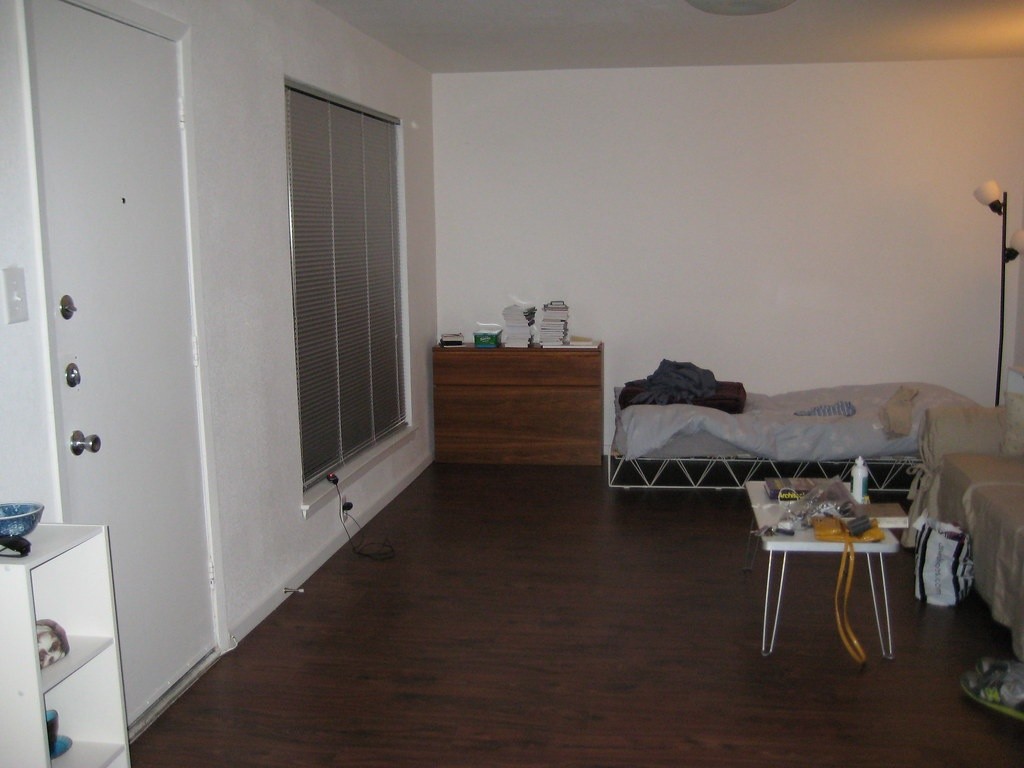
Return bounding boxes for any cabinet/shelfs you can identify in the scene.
[0,523,131,768]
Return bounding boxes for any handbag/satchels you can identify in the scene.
[911,508,976,607]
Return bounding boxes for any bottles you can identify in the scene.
[851,456,869,506]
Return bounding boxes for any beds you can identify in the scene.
[608,382,975,491]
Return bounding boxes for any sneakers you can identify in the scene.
[960,656,1024,722]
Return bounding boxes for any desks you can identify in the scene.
[745,481,900,659]
[432,343,604,465]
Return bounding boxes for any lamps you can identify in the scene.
[973,180,1024,407]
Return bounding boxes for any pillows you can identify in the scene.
[998,392,1024,456]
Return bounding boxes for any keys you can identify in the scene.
[750,527,776,536]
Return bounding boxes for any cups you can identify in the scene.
[46,710,58,753]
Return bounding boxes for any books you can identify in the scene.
[440,302,592,349]
[763,476,910,528]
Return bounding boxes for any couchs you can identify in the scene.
[900,392,1024,662]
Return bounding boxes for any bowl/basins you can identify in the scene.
[0,503,44,535]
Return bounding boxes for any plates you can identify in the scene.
[51,735,73,759]
